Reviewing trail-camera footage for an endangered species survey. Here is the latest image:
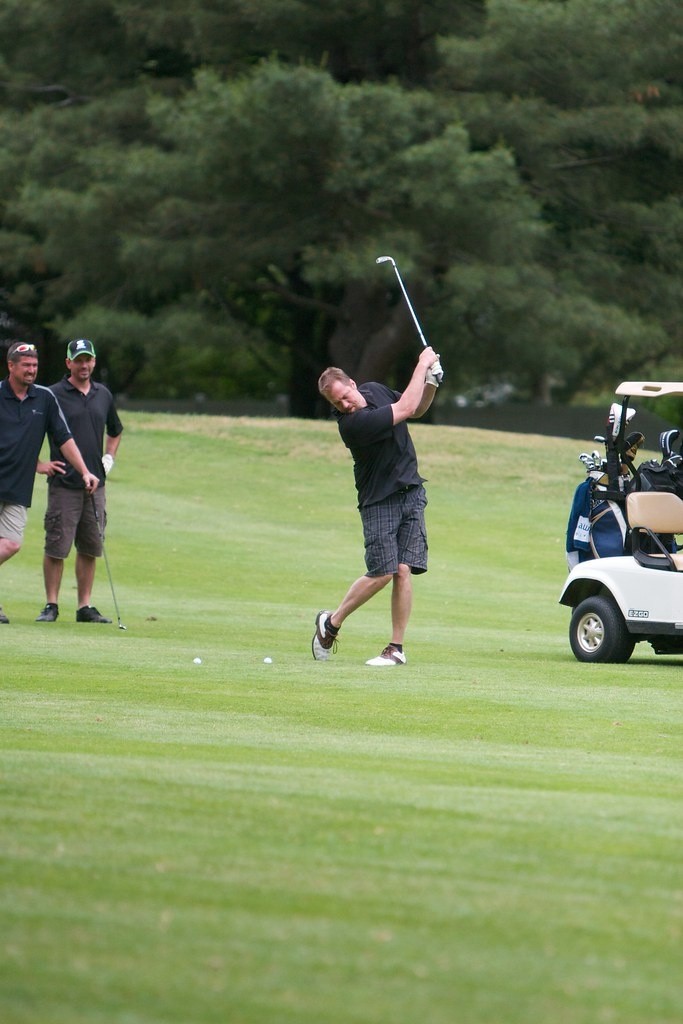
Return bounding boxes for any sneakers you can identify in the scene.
[35,604,59,621]
[76,606,112,623]
[312,610,339,661]
[0,606,9,624]
[365,645,406,666]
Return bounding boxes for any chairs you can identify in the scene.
[625,491,683,572]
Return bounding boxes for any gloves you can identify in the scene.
[102,454,114,475]
[425,354,443,387]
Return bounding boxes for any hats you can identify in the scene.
[67,339,95,360]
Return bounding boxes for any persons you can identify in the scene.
[36,339,124,624]
[311,345,445,666]
[0,339,99,622]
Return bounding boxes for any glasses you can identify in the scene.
[13,344,36,354]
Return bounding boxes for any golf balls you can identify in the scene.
[193,657,202,664]
[264,657,272,664]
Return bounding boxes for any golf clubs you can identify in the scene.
[89,481,127,630]
[578,435,624,478]
[375,255,444,385]
[659,429,683,470]
